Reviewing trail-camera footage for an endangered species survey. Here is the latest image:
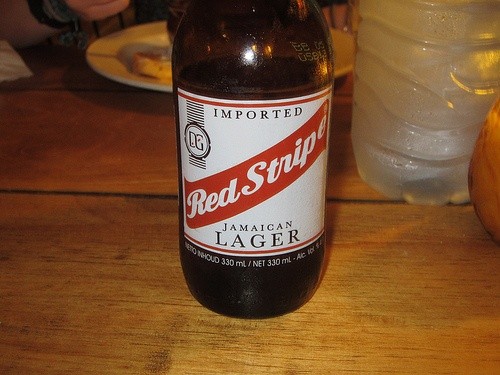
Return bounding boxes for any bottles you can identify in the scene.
[352,0,500,206]
[172,0,336,319]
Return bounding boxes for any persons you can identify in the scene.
[316,0,349,30]
[0,0,131,48]
[468,92,500,246]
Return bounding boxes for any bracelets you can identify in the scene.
[27,0,89,53]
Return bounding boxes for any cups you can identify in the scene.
[467,96,499,245]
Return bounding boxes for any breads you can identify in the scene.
[468,91,500,249]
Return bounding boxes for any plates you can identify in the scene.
[84,20,359,94]
